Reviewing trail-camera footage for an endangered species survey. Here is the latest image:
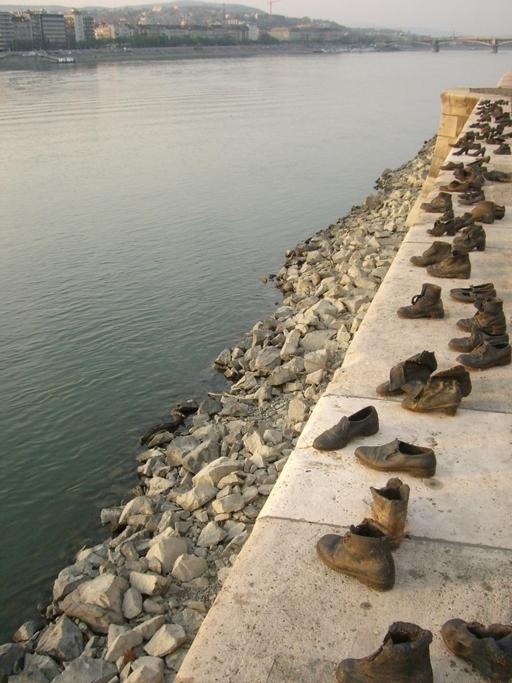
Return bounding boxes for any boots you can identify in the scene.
[409,241,452,267]
[427,247,471,279]
[376,350,472,416]
[396,284,445,320]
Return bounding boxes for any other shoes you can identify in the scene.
[493,144,510,155]
[439,162,508,191]
[463,98,512,143]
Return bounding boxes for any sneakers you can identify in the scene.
[316,517,396,592]
[368,478,410,551]
[335,620,434,683]
[440,618,512,682]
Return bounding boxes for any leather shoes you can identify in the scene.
[355,439,437,478]
[447,282,510,369]
[311,405,379,451]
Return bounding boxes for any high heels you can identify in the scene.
[448,143,490,162]
[420,190,505,251]
